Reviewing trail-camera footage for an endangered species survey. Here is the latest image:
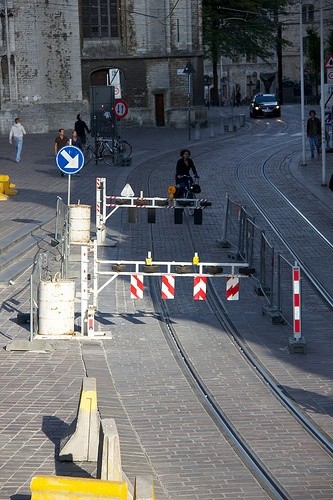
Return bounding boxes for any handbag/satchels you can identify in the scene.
[192,175,201,193]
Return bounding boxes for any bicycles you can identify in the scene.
[82,135,133,166]
[172,174,201,216]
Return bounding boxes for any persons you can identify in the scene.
[72,130,81,149]
[230,90,243,108]
[75,114,90,154]
[54,129,70,177]
[8,118,26,163]
[312,83,321,105]
[173,149,201,205]
[218,88,223,107]
[306,110,322,158]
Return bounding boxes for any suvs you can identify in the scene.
[250,92,282,118]
